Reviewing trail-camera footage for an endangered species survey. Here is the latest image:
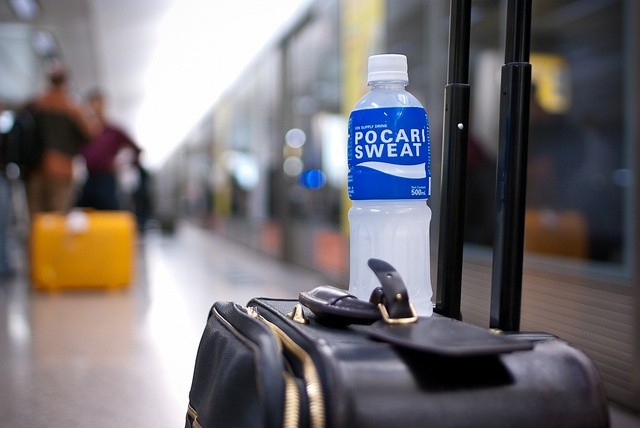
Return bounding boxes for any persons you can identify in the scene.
[8,62,102,214]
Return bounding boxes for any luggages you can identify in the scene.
[32,206,136,293]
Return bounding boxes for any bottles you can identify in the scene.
[348,53,433,318]
